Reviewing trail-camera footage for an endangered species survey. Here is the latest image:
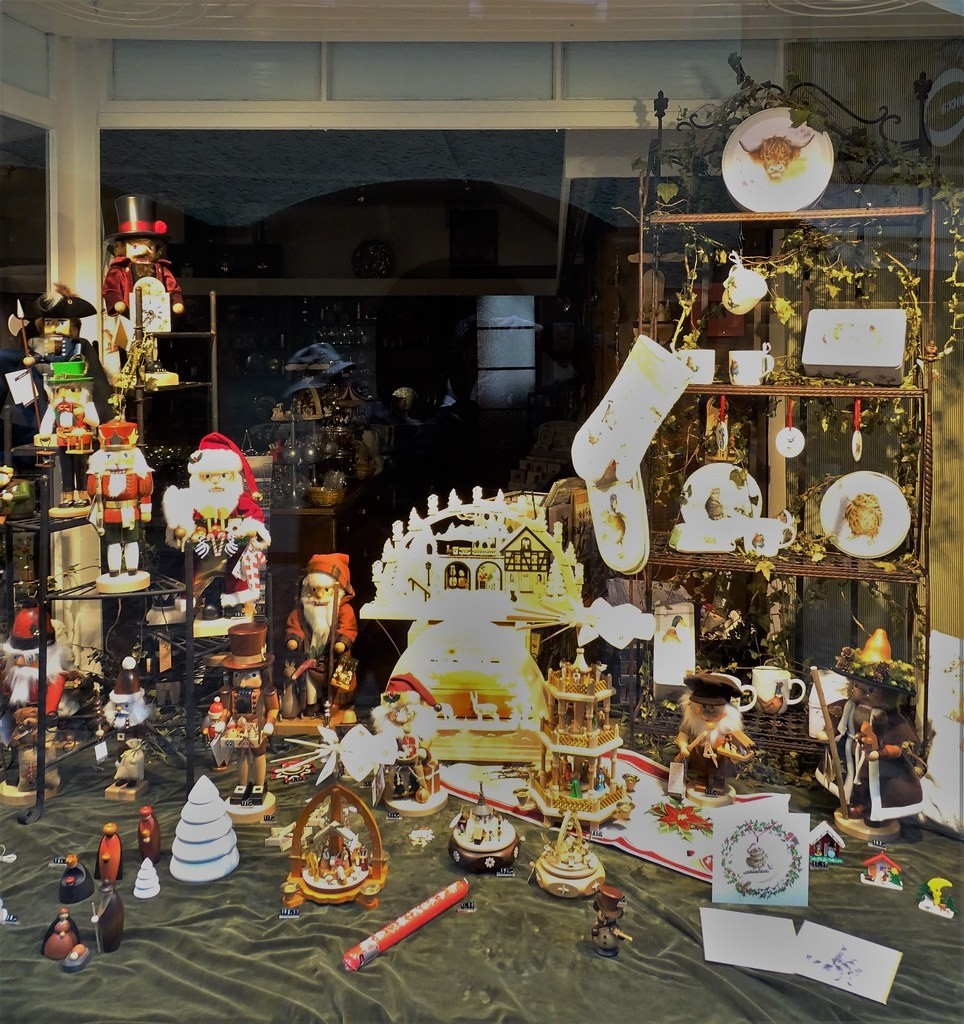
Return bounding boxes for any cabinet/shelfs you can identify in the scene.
[585,225,647,424]
[270,464,396,691]
[627,203,937,775]
[133,284,220,449]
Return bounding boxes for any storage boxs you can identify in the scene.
[806,303,913,386]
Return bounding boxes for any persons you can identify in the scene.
[285,553,357,717]
[0,604,69,787]
[40,362,100,509]
[203,622,279,804]
[677,674,743,798]
[87,420,154,578]
[102,194,184,372]
[163,432,271,621]
[591,886,625,957]
[24,296,98,394]
[104,657,149,788]
[815,628,925,826]
[372,674,442,802]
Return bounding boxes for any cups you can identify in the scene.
[742,516,796,558]
[728,350,774,387]
[680,350,715,383]
[752,666,806,714]
[722,265,769,316]
[712,672,756,714]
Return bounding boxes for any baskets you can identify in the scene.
[309,487,345,506]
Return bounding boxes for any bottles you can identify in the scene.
[114,656,146,763]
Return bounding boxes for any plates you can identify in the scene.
[680,462,764,523]
[820,472,912,560]
[721,107,835,213]
